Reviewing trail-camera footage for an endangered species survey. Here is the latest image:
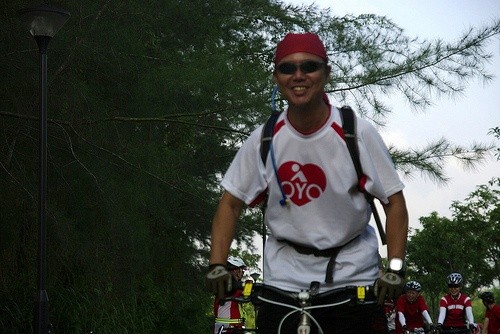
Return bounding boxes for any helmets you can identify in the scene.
[227,256,247,268]
[404,281,422,290]
[447,273,463,286]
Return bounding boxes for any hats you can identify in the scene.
[275,32,327,62]
[478,292,494,301]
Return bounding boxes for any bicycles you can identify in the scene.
[402,323,478,334]
[214,324,259,334]
[206,275,399,334]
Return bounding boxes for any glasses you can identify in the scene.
[277,60,321,74]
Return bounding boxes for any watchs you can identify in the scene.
[386,257,407,277]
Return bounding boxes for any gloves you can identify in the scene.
[374,271,404,305]
[203,264,233,306]
[473,323,482,332]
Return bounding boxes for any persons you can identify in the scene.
[385,273,500,334]
[205,33,409,334]
[213,255,247,334]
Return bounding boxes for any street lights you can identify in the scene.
[18,7,71,334]
